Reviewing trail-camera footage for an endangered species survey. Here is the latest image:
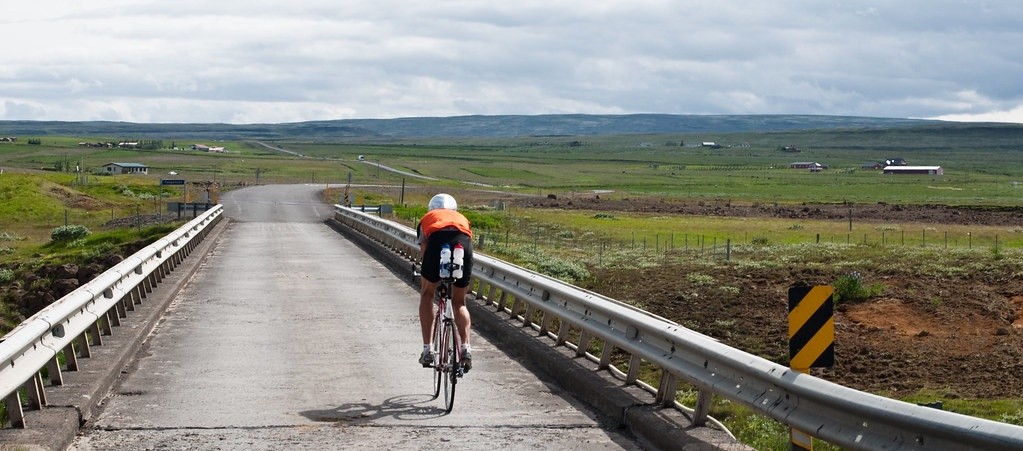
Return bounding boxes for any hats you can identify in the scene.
[428,193,457,210]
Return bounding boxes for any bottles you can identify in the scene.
[452,242,464,279]
[439,243,451,278]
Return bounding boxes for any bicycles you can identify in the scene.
[412,259,469,414]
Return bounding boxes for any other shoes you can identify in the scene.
[461,349,472,369]
[419,348,434,364]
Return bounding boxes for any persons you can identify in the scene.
[417,192,473,374]
